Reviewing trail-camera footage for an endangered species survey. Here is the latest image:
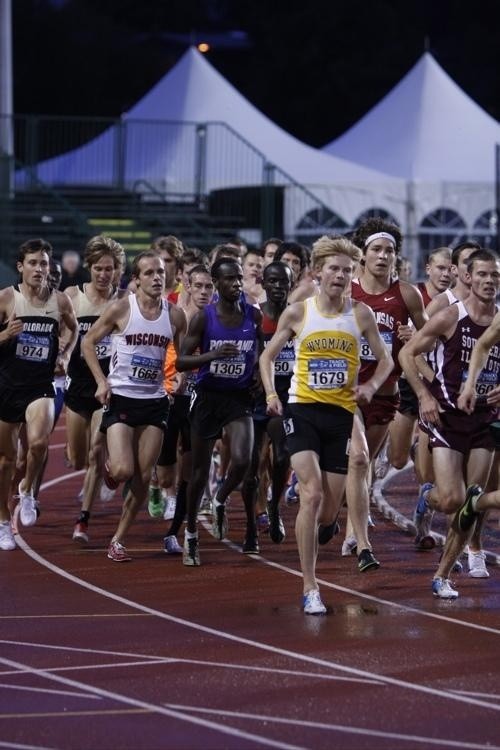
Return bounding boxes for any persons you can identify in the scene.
[1,212,500,613]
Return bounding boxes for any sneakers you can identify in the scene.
[432,578,459,599]
[13,477,41,527]
[302,584,327,615]
[413,484,498,578]
[2,519,17,552]
[64,453,381,573]
